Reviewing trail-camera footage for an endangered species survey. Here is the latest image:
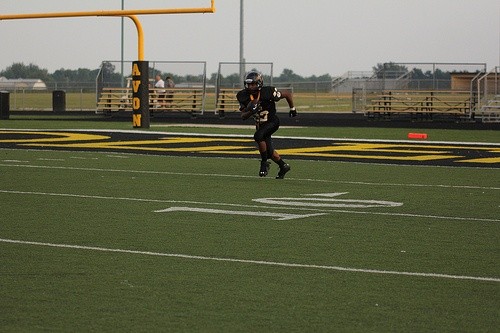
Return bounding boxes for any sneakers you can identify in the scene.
[276,164,290,180]
[259,161,270,177]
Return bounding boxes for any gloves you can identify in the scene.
[250,103,259,115]
[289,106,297,118]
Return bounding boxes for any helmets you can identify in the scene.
[244,72,263,92]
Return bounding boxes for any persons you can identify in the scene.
[154,75,165,108]
[236,72,296,180]
[164,77,174,112]
